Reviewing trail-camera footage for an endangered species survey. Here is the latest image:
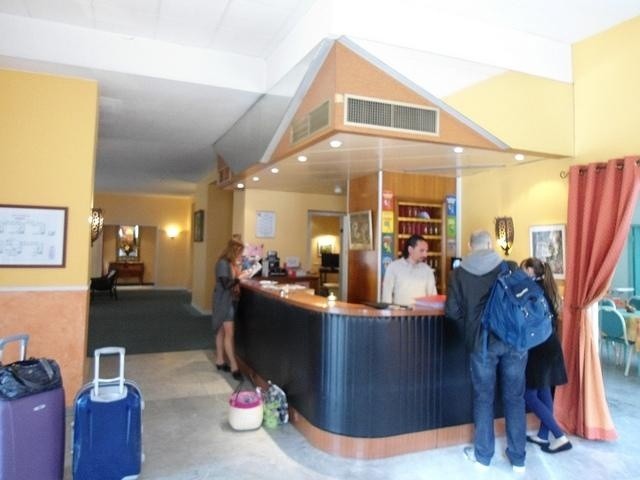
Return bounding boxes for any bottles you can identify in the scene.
[399,221,439,234]
[401,256,441,283]
[401,206,432,219]
[279,284,290,298]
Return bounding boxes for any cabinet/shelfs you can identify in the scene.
[394,198,447,294]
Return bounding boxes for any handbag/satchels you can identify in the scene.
[256,380,290,424]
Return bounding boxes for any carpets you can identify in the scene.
[86,289,216,357]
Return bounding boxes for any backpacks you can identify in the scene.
[479,259,553,366]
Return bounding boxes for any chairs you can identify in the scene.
[598,297,640,376]
[90,268,118,301]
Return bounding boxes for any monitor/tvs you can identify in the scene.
[321,253,339,270]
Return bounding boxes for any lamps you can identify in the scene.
[495,217,514,254]
[91,208,104,247]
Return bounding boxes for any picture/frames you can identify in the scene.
[348,210,373,251]
[530,225,566,280]
[0,204,68,267]
[193,210,203,242]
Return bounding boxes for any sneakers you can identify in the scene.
[504,448,525,473]
[462,446,490,467]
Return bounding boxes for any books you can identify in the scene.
[247,262,263,278]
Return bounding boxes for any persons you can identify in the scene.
[520,257,573,455]
[381,235,437,306]
[210,240,252,381]
[444,230,529,473]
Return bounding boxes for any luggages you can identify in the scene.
[1,334,66,480]
[70,346,145,480]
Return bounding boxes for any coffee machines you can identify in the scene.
[266,249,286,276]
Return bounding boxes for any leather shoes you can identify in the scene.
[216,362,231,372]
[233,369,243,380]
[526,436,550,447]
[541,441,572,453]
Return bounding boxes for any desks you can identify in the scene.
[319,267,339,287]
[109,262,144,284]
